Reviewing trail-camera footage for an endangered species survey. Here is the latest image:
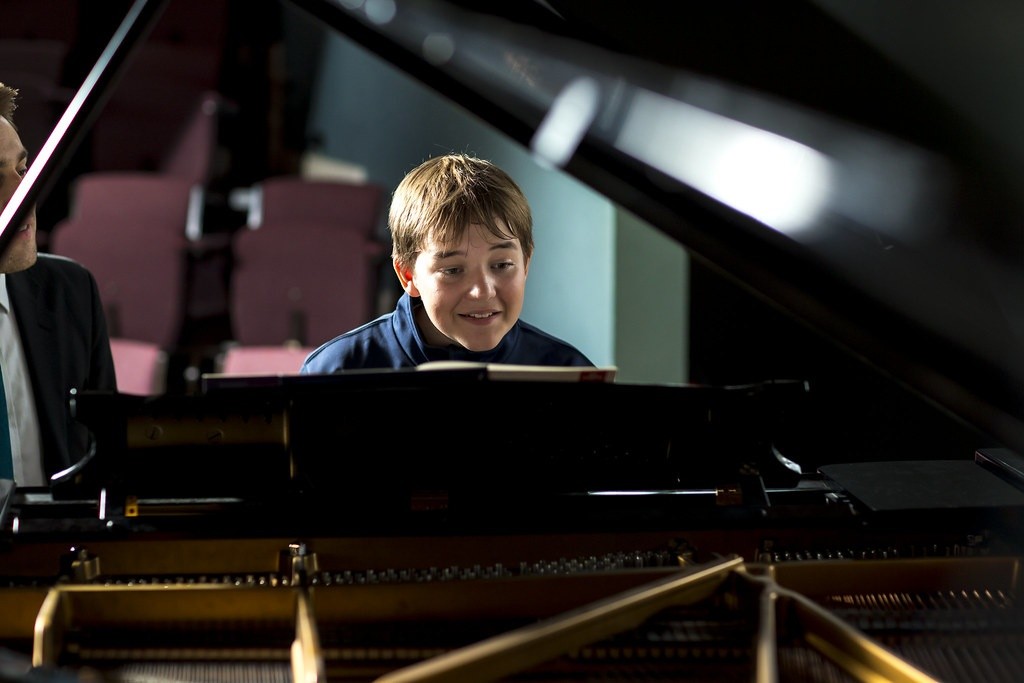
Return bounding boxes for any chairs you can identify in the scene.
[1,0,384,396]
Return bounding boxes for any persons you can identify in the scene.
[0,83,119,494]
[300,154,597,375]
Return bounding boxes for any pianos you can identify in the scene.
[0,0,1024,683]
